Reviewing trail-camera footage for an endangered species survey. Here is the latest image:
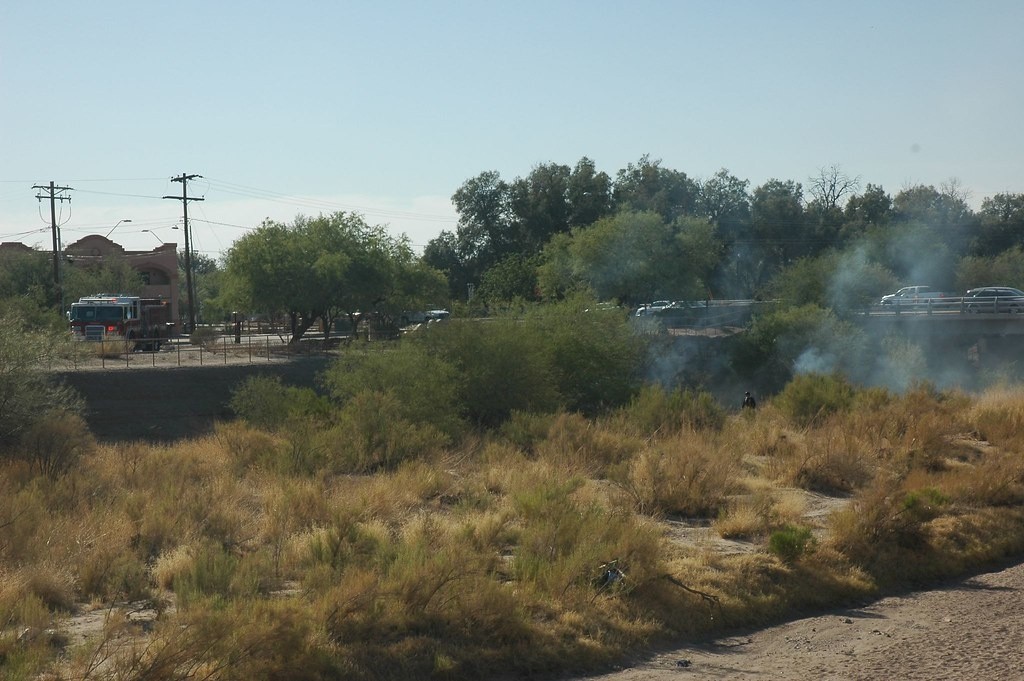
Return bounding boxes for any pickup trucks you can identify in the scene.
[880,285,950,309]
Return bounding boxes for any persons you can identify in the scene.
[741,391,756,410]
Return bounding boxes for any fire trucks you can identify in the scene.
[66,292,176,354]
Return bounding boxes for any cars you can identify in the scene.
[662,299,711,312]
[961,285,1024,314]
[635,300,671,320]
